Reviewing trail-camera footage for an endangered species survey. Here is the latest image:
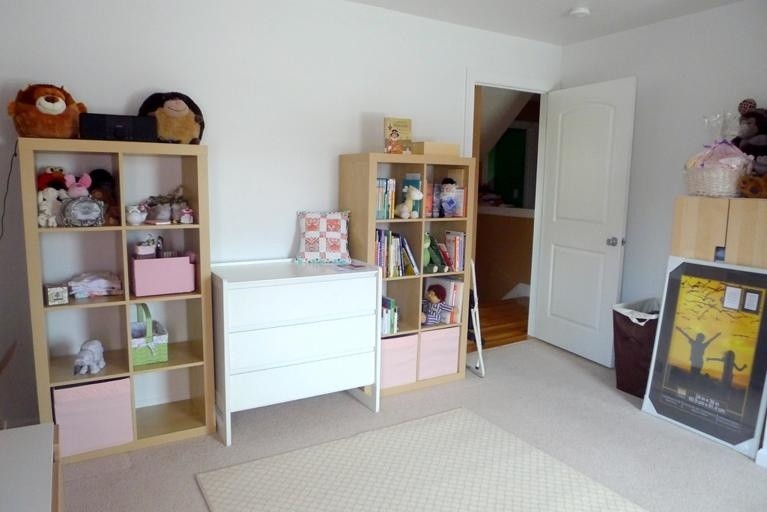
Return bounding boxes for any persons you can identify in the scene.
[706,349,748,386]
[675,325,722,373]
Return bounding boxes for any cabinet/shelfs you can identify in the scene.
[211,257,385,447]
[338,153,477,397]
[17,137,216,463]
[671,196,767,270]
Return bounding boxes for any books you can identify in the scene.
[374,174,466,337]
[384,116,412,154]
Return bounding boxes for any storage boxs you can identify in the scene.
[381,334,416,389]
[129,256,195,297]
[419,327,460,380]
[52,378,133,458]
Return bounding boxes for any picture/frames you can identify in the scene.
[641,255,767,460]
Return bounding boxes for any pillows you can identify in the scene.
[297,211,353,263]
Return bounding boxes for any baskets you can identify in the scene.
[686,159,748,198]
[131,302,168,366]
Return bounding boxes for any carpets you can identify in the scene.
[196,408,650,512]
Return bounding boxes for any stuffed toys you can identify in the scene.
[731,107,767,177]
[132,92,205,144]
[34,164,122,228]
[7,82,88,140]
[72,338,107,375]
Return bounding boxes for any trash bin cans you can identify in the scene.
[611,298,665,399]
